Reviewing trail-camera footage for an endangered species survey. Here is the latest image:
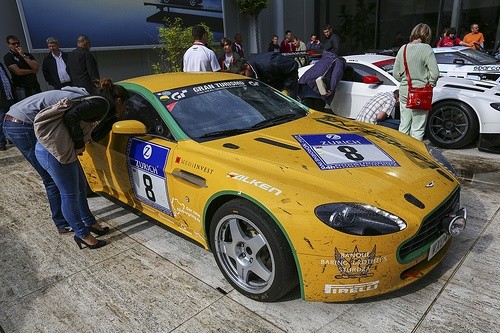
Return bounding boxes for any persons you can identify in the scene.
[291,35,308,68]
[232,34,244,60]
[217,37,241,72]
[42,36,74,90]
[268,35,280,52]
[3,35,43,103]
[437,27,475,47]
[297,48,347,111]
[34,77,130,250]
[463,23,485,52]
[229,51,299,101]
[354,89,401,130]
[280,30,296,53]
[0,61,19,151]
[322,24,341,56]
[393,23,439,142]
[306,34,322,53]
[65,33,100,89]
[182,26,222,72]
[2,80,99,232]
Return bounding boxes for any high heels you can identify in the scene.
[74,235,107,249]
[87,226,110,236]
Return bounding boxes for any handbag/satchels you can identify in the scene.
[315,76,330,95]
[405,85,433,110]
[33,96,110,164]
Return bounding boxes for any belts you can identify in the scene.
[5,115,30,125]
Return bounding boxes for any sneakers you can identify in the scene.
[59,226,73,233]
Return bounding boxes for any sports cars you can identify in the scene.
[432,46,500,82]
[76,71,468,302]
[297,55,500,153]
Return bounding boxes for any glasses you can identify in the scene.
[9,42,20,45]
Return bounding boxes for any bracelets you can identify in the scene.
[22,54,30,62]
